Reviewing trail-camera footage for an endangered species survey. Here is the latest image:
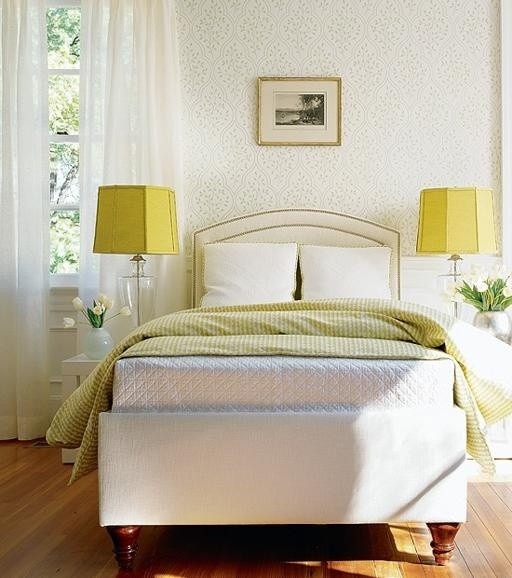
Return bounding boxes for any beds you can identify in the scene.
[97,207,468,568]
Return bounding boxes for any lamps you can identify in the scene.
[416,187,499,317]
[92,185,180,326]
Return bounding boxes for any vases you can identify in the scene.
[84,329,115,360]
[474,312,511,344]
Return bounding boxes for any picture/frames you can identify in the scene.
[258,76,342,146]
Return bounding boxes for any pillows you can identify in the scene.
[199,242,299,307]
[298,244,397,301]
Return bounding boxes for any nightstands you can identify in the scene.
[62,353,102,463]
[468,415,512,459]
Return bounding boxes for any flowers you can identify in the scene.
[62,293,133,327]
[439,264,512,310]
